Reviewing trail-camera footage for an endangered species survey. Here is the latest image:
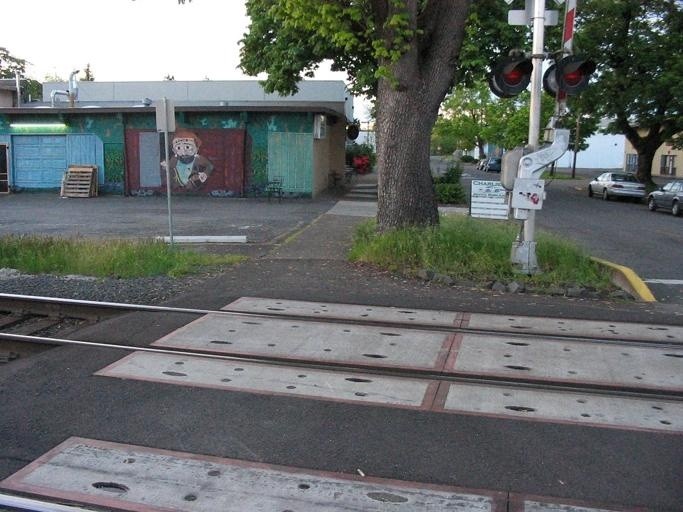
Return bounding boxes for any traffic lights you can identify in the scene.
[495,58,533,93]
[556,57,595,93]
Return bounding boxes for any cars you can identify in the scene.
[647,179,683,217]
[588,172,645,202]
[477,157,501,173]
